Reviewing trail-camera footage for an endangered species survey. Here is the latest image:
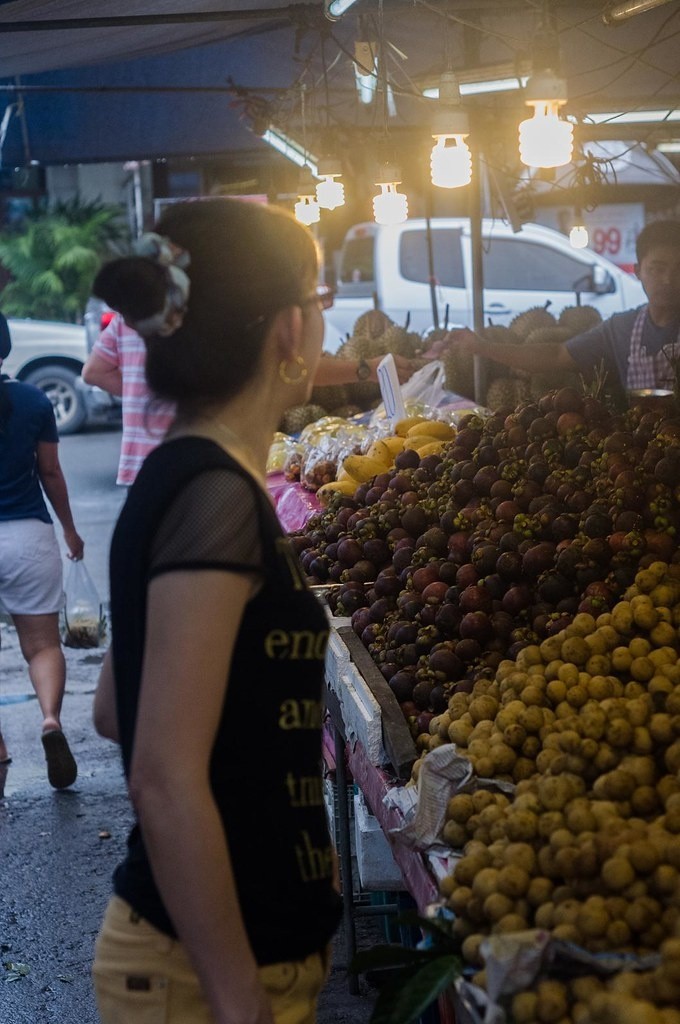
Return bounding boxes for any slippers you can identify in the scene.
[40,728,78,791]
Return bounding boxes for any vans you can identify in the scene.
[84,284,351,426]
[334,215,651,358]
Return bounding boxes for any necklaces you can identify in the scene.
[175,402,263,473]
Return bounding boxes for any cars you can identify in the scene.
[0,316,123,439]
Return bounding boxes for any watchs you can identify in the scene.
[357,357,371,384]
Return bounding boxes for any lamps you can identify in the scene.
[568,206,588,249]
[315,34,347,212]
[429,14,474,188]
[372,107,409,226]
[293,82,320,226]
[517,1,574,169]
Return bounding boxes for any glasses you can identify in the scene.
[240,282,335,332]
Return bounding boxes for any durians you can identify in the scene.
[282,292,604,435]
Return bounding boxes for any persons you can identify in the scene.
[78,310,414,481]
[0,315,89,800]
[89,199,340,1023]
[442,218,679,417]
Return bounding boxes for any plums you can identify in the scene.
[405,561,680,1024]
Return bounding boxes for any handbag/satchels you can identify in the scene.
[56,557,110,652]
